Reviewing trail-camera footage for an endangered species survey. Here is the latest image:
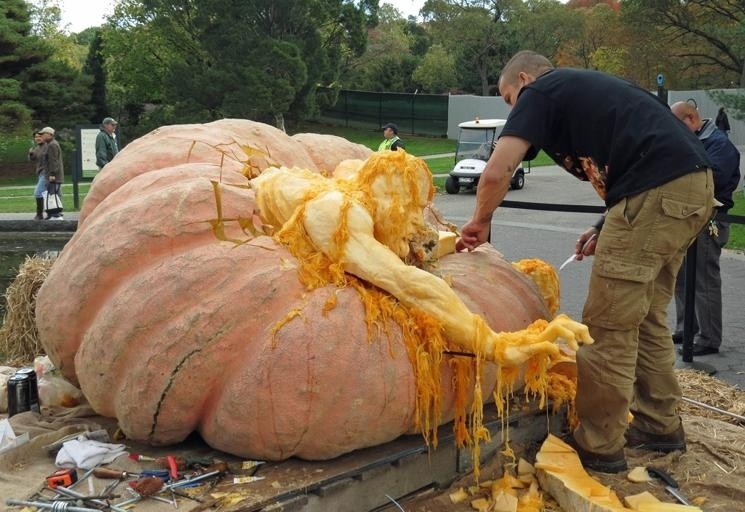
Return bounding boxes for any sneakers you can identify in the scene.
[671,333,718,355]
[624,421,686,453]
[562,433,627,473]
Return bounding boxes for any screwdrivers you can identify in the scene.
[93,468,139,479]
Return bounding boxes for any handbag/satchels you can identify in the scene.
[42,190,63,210]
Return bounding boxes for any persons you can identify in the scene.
[669,101,742,356]
[27,129,51,218]
[454,50,715,474]
[376,123,406,153]
[94,117,120,168]
[716,106,731,137]
[41,127,65,219]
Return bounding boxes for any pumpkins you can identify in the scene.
[34,118,705,512]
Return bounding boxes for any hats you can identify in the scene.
[38,127,55,135]
[103,118,118,125]
[382,123,399,134]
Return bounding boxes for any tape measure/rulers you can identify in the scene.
[46,467,78,489]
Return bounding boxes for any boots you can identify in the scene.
[33,197,43,219]
